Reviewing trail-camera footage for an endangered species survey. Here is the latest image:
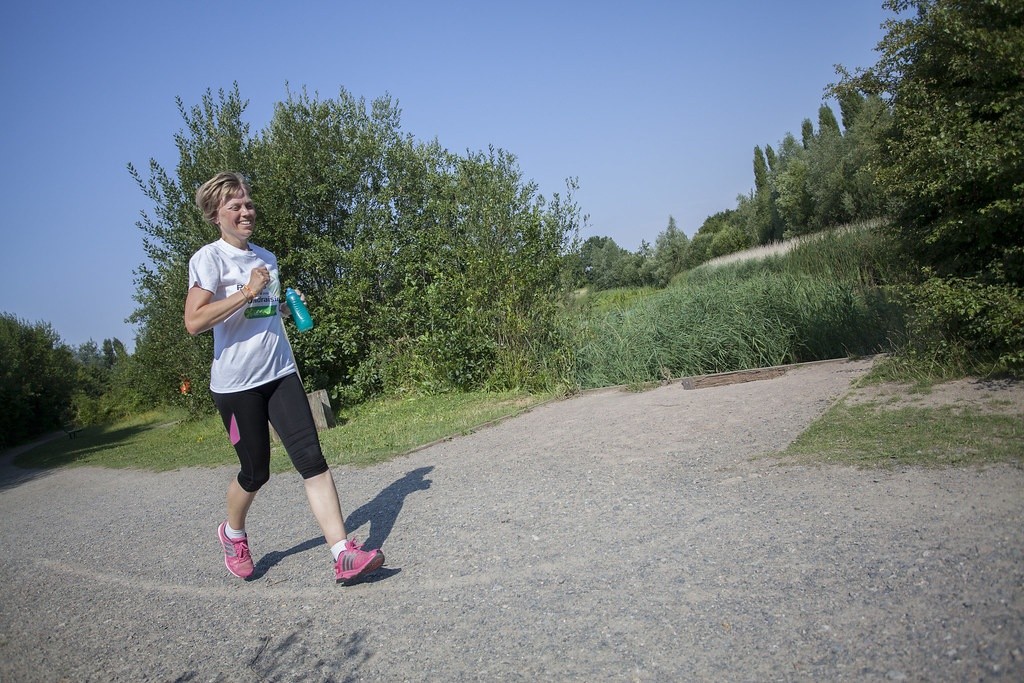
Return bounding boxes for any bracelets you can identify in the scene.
[240,290,251,303]
[244,285,256,297]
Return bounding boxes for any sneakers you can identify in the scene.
[335,537,385,587]
[218,521,255,578]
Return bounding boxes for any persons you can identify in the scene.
[184,172,385,586]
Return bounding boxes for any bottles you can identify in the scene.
[285,287,313,331]
